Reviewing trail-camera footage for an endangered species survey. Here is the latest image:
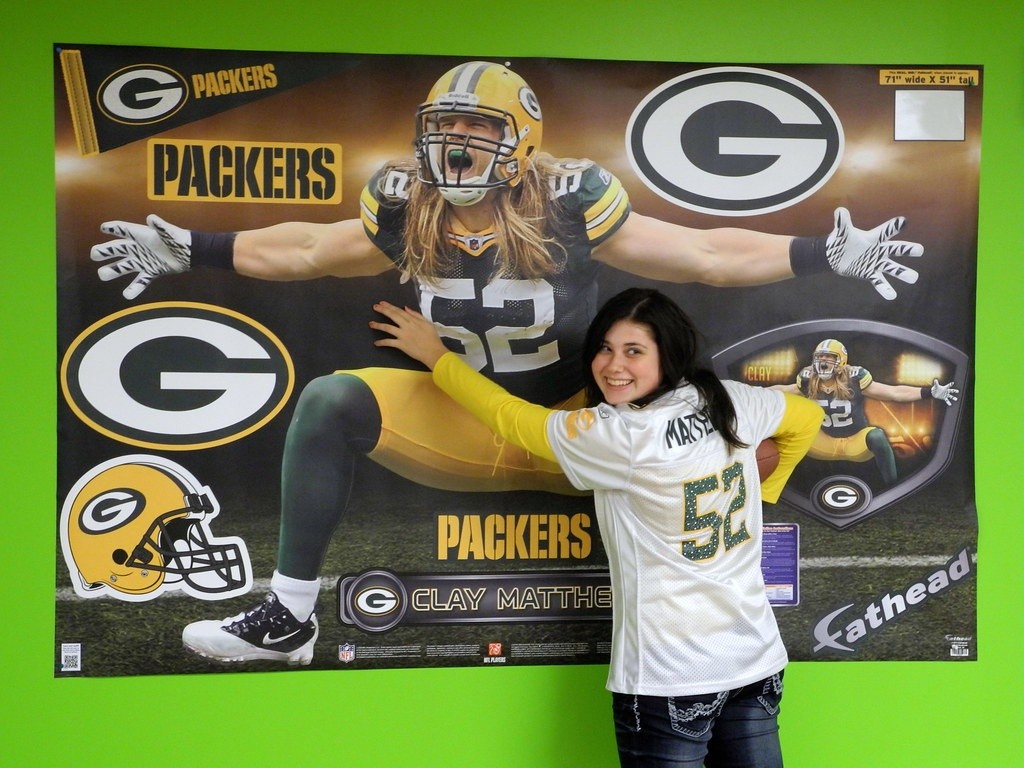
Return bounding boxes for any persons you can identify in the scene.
[764,338,960,487]
[369,287,826,768]
[89,61,926,665]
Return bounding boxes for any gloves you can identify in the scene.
[90,214,193,299]
[825,206,924,299]
[931,379,959,405]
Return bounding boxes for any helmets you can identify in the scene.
[422,61,543,206]
[813,339,848,381]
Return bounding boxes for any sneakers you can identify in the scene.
[183,590,317,667]
